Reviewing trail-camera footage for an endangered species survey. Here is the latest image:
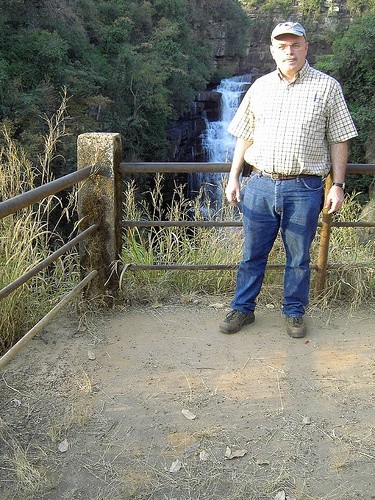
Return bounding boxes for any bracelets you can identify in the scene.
[331,182,345,189]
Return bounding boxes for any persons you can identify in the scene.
[220,21,359,339]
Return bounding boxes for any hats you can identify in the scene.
[272,22,306,38]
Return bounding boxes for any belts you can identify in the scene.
[253,167,309,181]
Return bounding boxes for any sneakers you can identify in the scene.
[286,315,306,337]
[218,309,255,334]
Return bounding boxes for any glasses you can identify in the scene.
[273,41,305,50]
